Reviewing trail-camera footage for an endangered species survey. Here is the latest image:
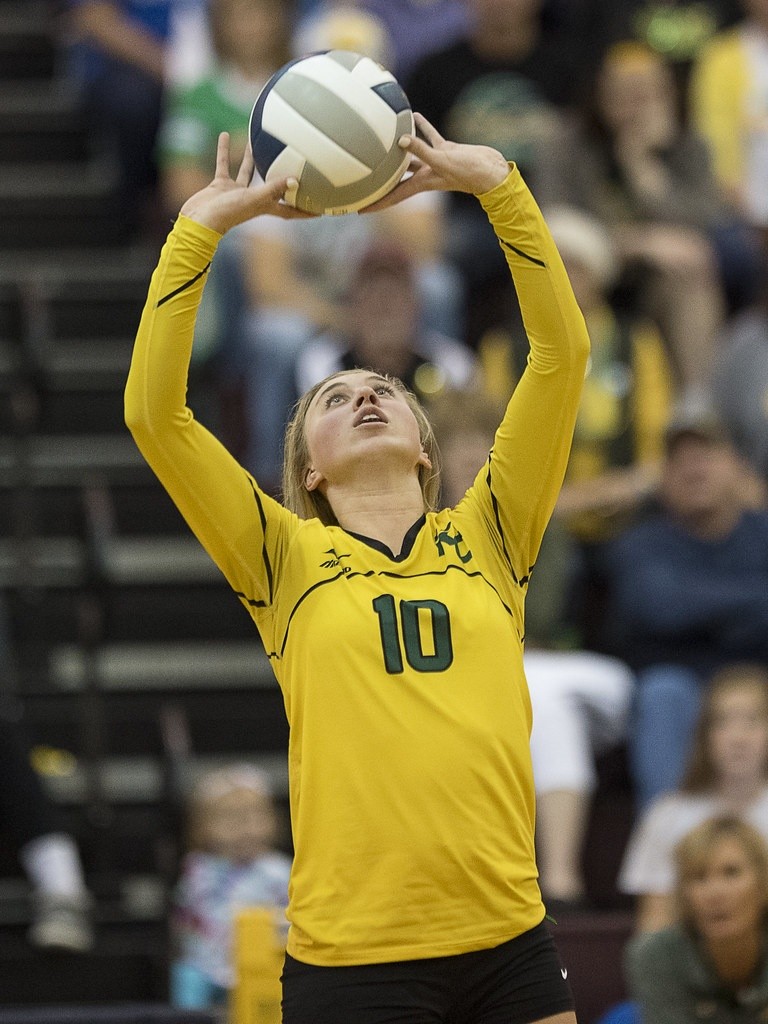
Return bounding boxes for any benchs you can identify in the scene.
[0,0,288,1024]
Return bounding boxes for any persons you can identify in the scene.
[0,0,768,1024]
[122,109,588,1024]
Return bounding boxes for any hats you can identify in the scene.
[663,394,727,450]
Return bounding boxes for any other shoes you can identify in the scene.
[25,895,104,967]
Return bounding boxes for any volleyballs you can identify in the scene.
[247,49,416,217]
